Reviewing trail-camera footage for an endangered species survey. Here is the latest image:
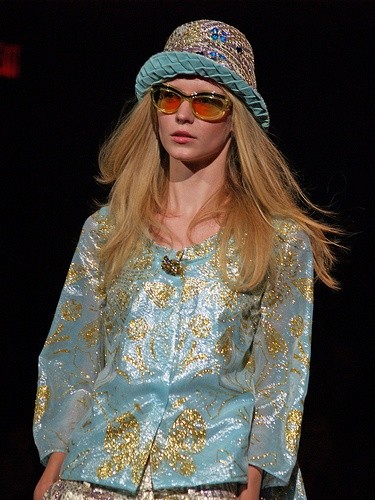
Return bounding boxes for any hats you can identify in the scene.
[133,19,270,133]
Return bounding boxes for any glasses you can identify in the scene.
[150,81,233,122]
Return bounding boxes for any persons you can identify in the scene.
[32,19,347,500]
